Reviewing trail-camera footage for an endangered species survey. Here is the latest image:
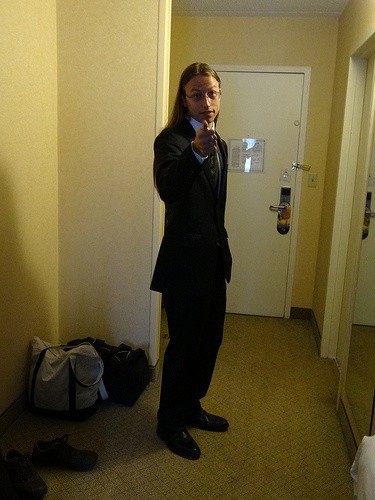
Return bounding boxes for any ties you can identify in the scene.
[203,147,218,192]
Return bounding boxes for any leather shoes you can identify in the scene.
[184,407,229,432]
[156,424,201,461]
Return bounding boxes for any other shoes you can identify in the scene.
[5,447,47,497]
[31,434,97,472]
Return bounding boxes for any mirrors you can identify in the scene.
[344,52,375,442]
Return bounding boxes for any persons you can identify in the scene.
[150,62,232,461]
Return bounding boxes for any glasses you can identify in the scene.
[185,90,221,101]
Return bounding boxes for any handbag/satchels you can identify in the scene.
[27,336,104,422]
[62,338,150,407]
